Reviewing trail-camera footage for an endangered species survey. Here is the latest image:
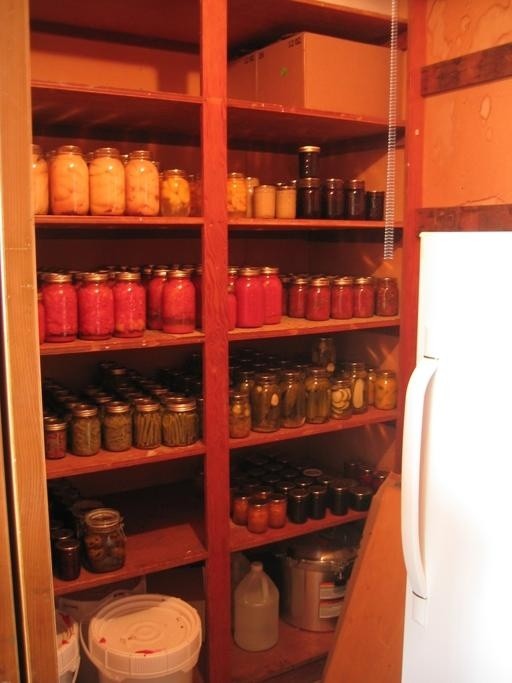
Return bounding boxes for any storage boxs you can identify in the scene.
[229,31,406,123]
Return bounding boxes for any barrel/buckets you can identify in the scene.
[58,575,148,681]
[55,609,82,683]
[233,561,280,652]
[79,589,203,683]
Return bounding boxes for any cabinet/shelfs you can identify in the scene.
[0,0,510,682]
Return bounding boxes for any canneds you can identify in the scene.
[43,351,204,460]
[226,145,385,221]
[228,336,398,438]
[48,479,127,581]
[230,450,388,534]
[31,144,201,217]
[227,264,399,331]
[37,263,203,346]
